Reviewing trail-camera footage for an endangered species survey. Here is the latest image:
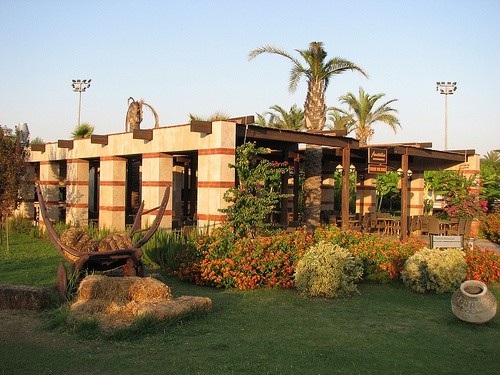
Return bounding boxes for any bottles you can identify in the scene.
[451,280,496,324]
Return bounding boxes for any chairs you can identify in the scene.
[319,206,474,242]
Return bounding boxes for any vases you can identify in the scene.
[451,279,497,325]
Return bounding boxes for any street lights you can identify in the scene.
[71,79,91,127]
[435,81,457,151]
